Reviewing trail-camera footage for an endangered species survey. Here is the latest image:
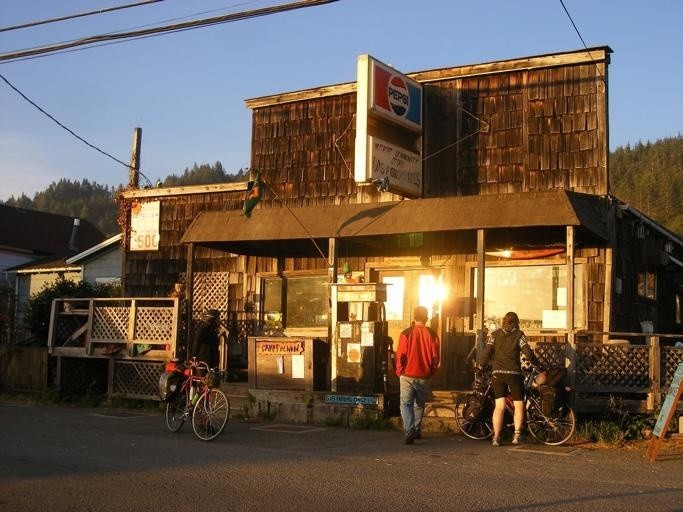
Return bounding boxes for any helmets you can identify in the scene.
[199,309,220,323]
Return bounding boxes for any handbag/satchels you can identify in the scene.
[160,370,181,402]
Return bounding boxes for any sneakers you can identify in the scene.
[405,429,420,444]
[512,435,524,444]
[492,438,504,446]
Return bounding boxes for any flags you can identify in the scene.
[239,169,270,217]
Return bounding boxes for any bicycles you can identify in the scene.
[164,356,231,442]
[454,359,576,446]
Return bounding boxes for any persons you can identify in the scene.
[189,311,225,437]
[482,312,545,446]
[394,306,441,444]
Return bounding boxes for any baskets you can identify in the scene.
[200,371,222,390]
